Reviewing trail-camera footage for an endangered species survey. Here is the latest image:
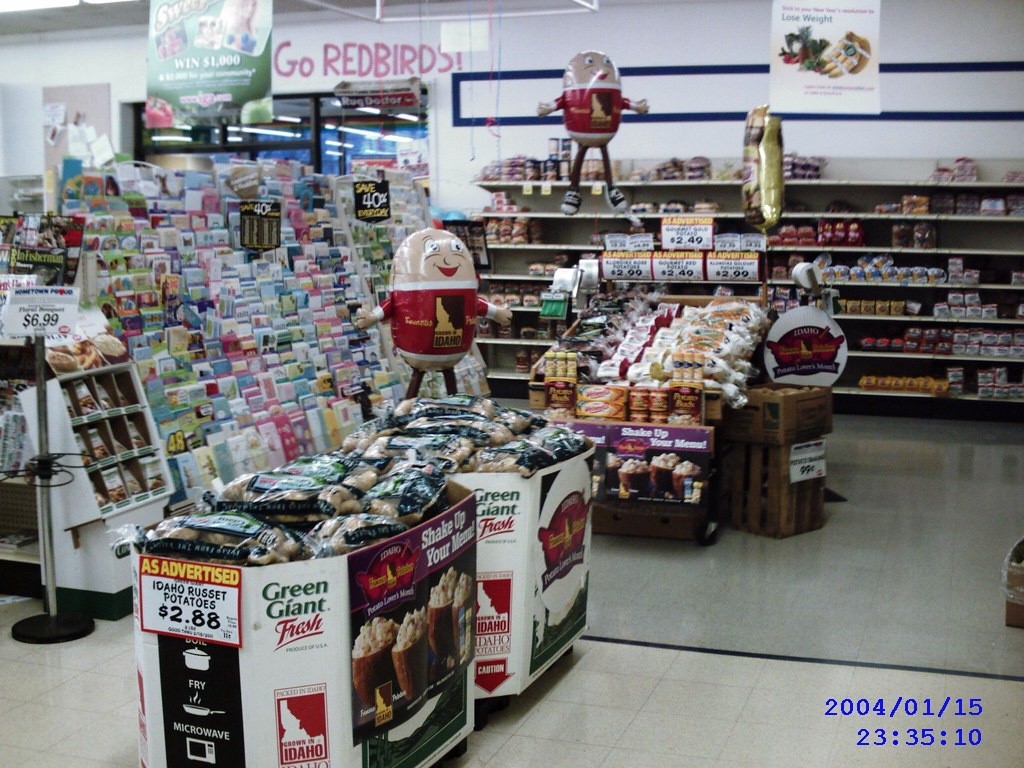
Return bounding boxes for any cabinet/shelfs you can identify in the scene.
[525,281,767,547]
[462,180,1024,422]
[0,337,176,622]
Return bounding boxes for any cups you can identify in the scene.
[671,472,702,500]
[426,601,456,658]
[352,638,396,709]
[619,471,650,496]
[391,625,430,700]
[452,595,471,649]
[648,464,675,490]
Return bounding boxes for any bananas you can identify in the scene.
[813,31,871,78]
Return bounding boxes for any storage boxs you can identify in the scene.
[1000,537,1024,630]
[720,382,834,445]
[714,437,827,540]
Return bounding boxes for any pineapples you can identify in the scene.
[799,46,812,65]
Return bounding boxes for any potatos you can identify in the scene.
[153,396,586,566]
